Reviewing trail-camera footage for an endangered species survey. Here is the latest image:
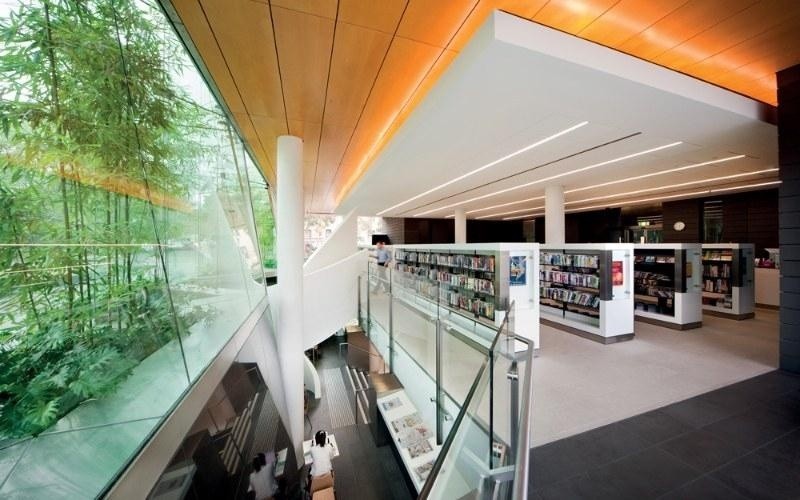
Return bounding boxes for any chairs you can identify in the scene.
[304,472,334,500]
[304,393,312,431]
[310,473,335,500]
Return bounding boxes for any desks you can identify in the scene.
[243,447,290,494]
[377,387,471,500]
[303,434,339,479]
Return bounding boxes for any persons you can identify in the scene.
[371,242,392,296]
[304,431,333,492]
[248,451,277,500]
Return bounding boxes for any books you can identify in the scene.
[633,253,674,316]
[394,249,496,322]
[383,396,402,410]
[702,249,733,309]
[538,252,600,311]
[416,459,447,482]
[391,412,434,458]
